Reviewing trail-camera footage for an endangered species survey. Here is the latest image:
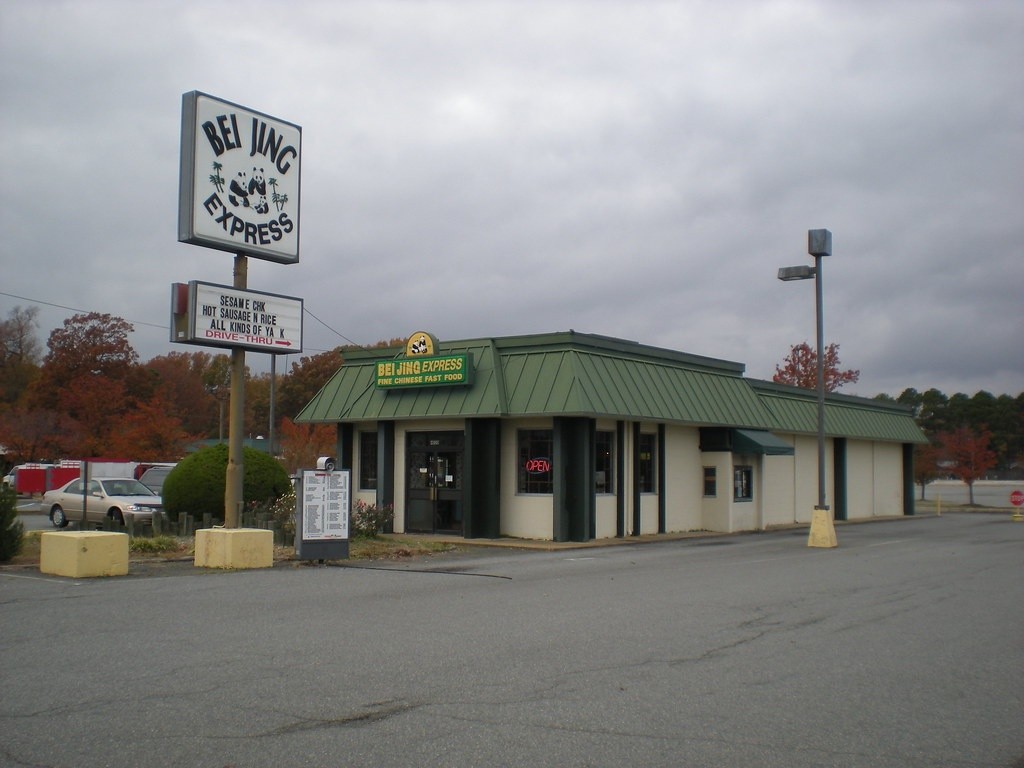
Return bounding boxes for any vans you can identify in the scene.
[2,463,60,494]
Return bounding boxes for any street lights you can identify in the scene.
[774,226,834,511]
[204,383,231,442]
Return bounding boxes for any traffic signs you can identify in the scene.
[185,279,305,356]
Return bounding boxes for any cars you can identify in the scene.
[39,475,166,530]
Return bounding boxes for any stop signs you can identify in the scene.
[1009,489,1023,507]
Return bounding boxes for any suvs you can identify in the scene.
[130,465,176,497]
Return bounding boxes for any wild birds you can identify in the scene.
[91,369,101,375]
